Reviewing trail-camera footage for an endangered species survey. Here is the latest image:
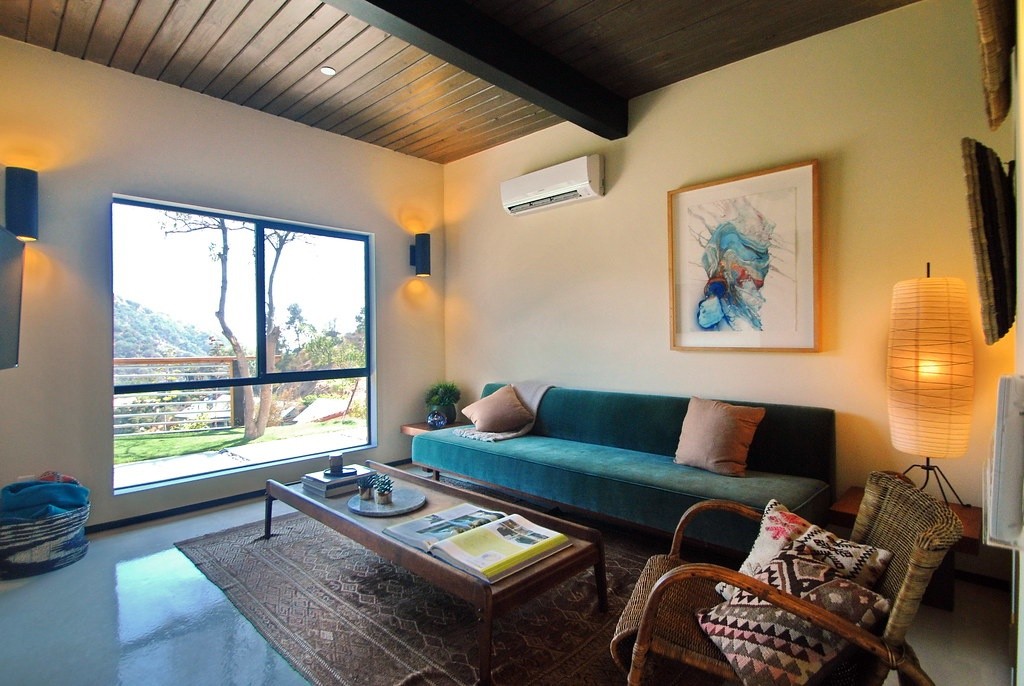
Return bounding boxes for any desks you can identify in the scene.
[401,424,462,436]
[831,487,983,613]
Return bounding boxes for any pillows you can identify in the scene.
[462,385,535,433]
[674,396,766,478]
[715,497,892,600]
[694,541,891,685]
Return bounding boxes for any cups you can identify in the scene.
[329,451,343,473]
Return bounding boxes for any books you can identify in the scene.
[382,503,573,585]
[301,464,377,499]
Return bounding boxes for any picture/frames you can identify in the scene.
[668,160,820,351]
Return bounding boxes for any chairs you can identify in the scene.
[608,471,963,686]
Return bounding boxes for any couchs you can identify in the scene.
[412,382,834,550]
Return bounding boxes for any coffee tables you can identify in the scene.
[263,459,607,686]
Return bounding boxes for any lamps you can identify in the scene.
[410,233,431,276]
[5,166,38,241]
[886,261,977,510]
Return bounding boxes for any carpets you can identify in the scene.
[172,474,666,685]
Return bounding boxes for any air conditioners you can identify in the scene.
[502,154,605,217]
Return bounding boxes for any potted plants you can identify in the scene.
[424,382,460,423]
